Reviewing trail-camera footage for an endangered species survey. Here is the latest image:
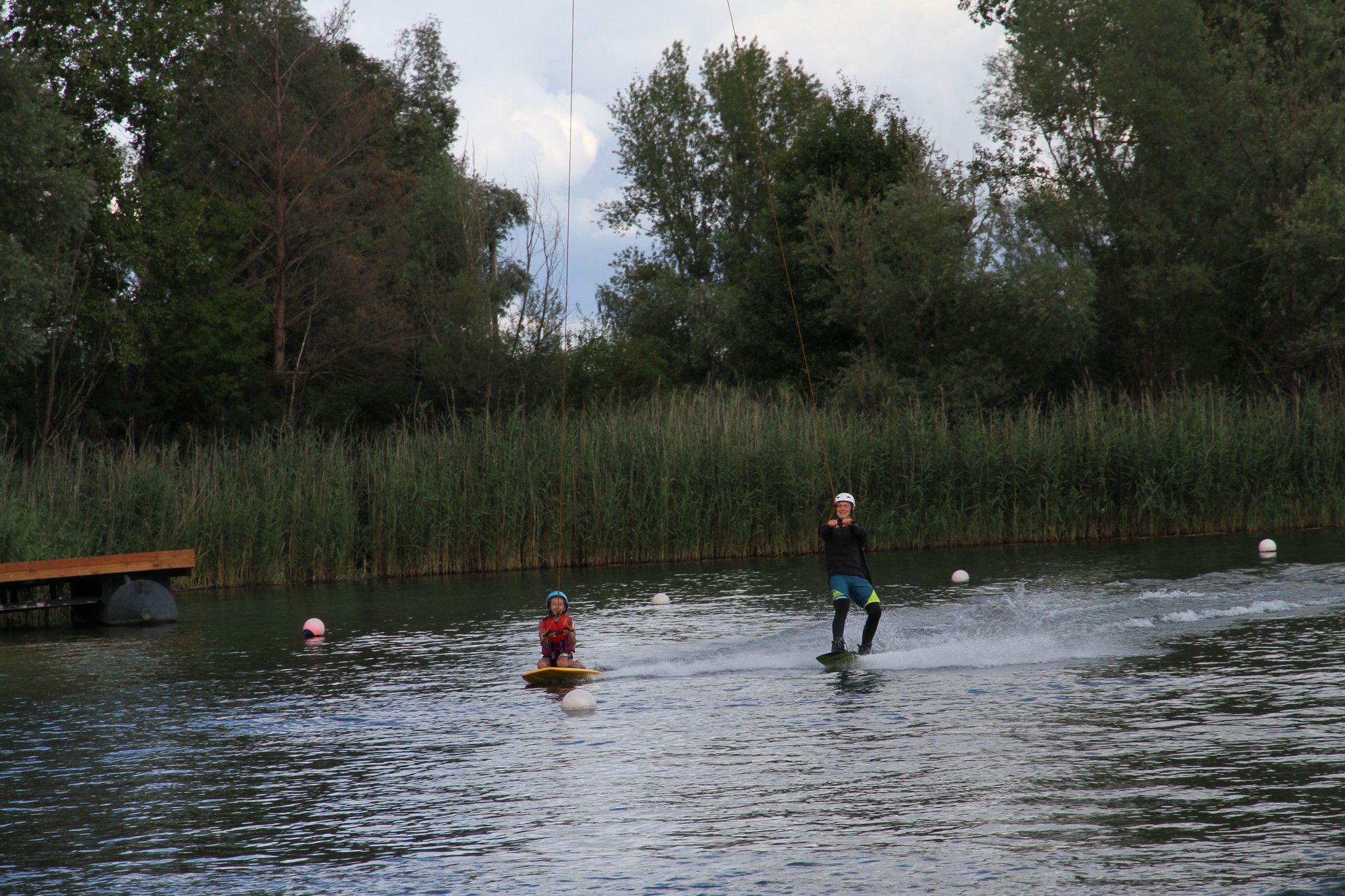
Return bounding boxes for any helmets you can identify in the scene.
[834,493,856,514]
[546,591,569,613]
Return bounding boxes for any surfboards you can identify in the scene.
[815,650,864,669]
[521,666,604,684]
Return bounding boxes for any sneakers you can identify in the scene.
[831,636,849,652]
[857,643,873,656]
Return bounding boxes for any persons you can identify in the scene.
[819,493,883,655]
[537,591,586,669]
